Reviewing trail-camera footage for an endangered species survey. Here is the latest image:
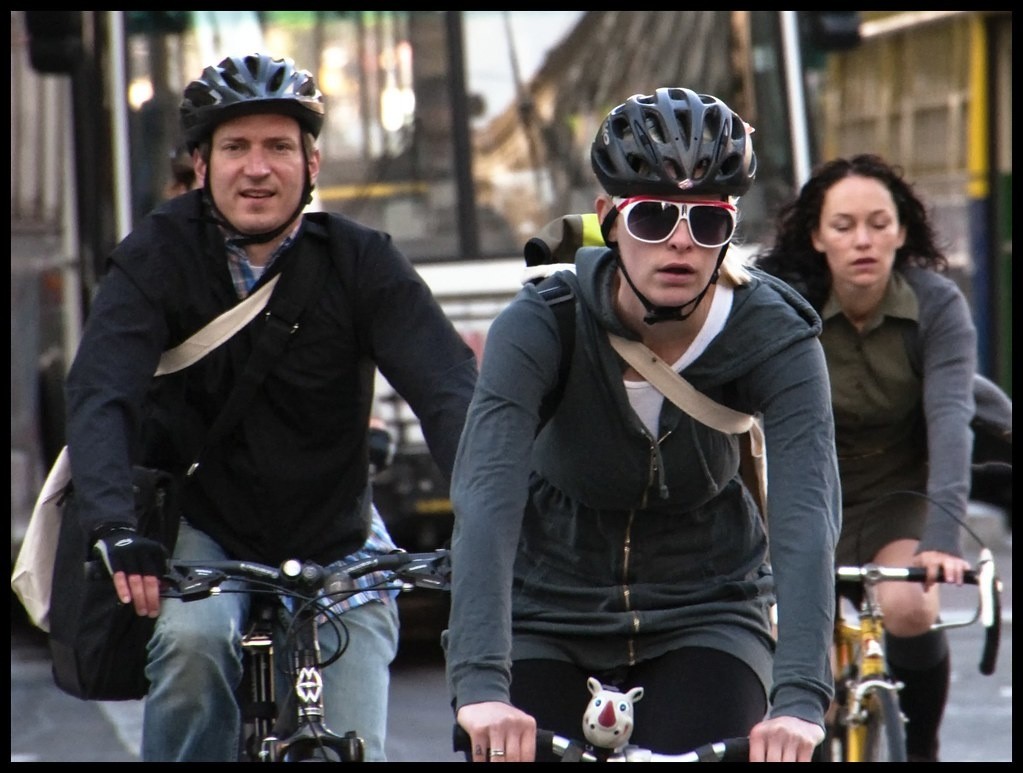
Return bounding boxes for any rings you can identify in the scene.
[490,748,504,757]
[471,744,486,755]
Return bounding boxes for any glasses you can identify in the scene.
[607,197,738,248]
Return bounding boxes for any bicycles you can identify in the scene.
[762,490,1005,764]
[84,550,454,763]
[452,676,832,763]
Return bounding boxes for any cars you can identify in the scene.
[371,238,1012,668]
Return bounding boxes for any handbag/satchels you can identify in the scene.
[11,441,70,632]
[49,464,180,701]
[522,210,770,523]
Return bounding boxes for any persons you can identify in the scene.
[62,52,978,761]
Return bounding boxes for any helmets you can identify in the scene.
[180,54,324,153]
[590,86,757,195]
